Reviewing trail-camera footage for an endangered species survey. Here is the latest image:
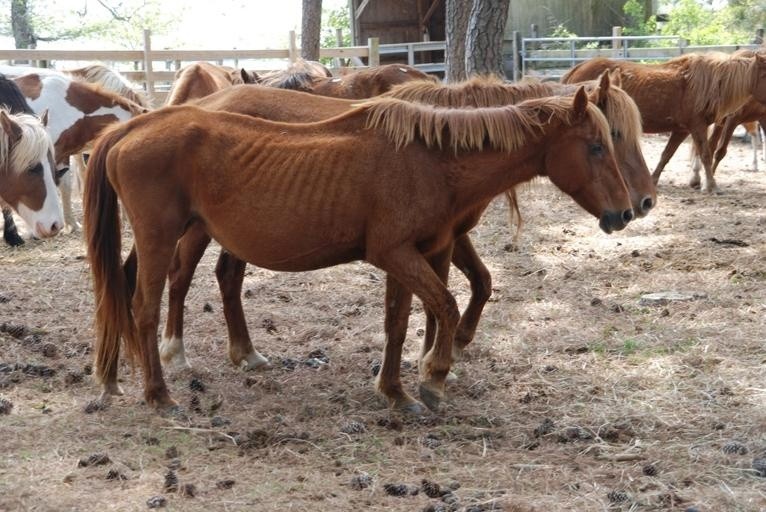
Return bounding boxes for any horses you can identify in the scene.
[163,60,438,107]
[82,85,635,416]
[560,44,766,195]
[159,67,657,384]
[0,63,157,248]
